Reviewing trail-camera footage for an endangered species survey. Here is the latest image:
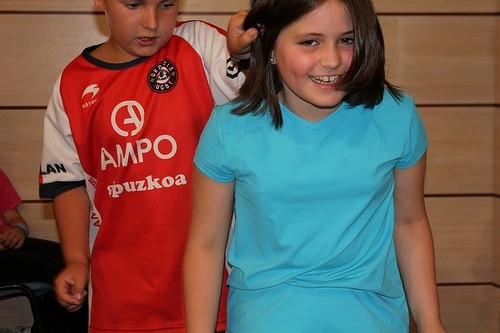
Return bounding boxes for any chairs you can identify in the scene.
[0,283,42,333]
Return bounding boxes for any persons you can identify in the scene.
[39,0,258,333]
[183,0,446,333]
[0,168,29,250]
